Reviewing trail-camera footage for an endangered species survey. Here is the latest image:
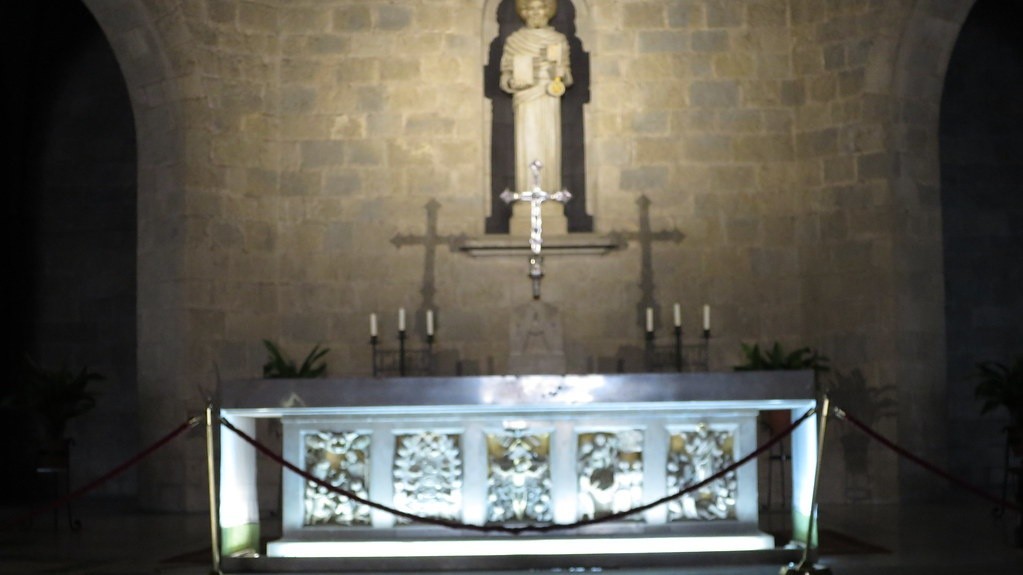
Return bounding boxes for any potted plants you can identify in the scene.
[973,356,1023,456]
[734,339,833,435]
[0,352,107,433]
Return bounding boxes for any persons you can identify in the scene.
[488,455,551,523]
[667,425,736,522]
[499,0,574,233]
[580,435,646,519]
[308,434,368,524]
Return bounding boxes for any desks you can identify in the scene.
[209,368,827,575]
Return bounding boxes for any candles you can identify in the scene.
[398,307,405,331]
[369,313,378,337]
[426,309,434,335]
[673,302,681,326]
[646,307,653,332]
[703,303,710,330]
